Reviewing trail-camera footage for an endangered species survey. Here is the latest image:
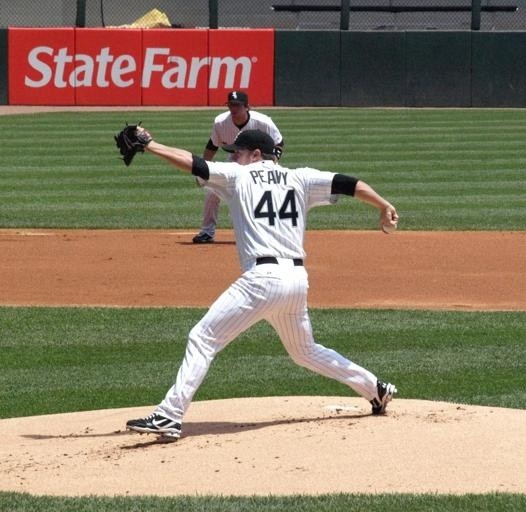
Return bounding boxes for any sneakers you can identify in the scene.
[371,379,398,416]
[124,414,183,440]
[191,231,213,243]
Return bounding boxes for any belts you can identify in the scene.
[256,256,304,267]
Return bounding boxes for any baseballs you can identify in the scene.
[383,221,397,234]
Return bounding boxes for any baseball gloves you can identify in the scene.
[114,124,152,167]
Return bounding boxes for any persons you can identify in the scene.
[192,90,284,243]
[113,120,398,437]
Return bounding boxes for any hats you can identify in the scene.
[225,90,249,105]
[221,129,275,156]
